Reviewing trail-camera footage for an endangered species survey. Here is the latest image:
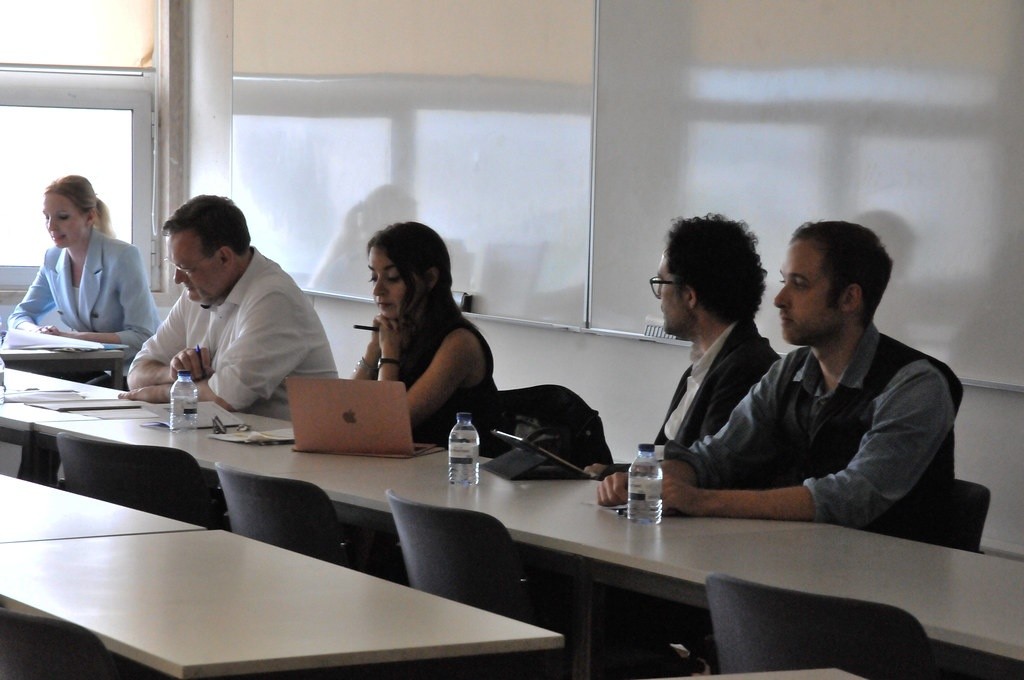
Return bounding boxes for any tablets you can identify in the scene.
[494,429,591,477]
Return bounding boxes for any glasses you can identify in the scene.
[650,277,674,299]
[164,242,230,275]
[212,416,226,435]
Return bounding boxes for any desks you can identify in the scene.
[0,348,1024,680]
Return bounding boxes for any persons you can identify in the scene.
[7,174,163,489]
[350,222,512,460]
[119,195,339,530]
[597,220,964,546]
[585,212,782,480]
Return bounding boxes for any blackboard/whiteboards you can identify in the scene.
[232,0,596,332]
[588,0,1024,393]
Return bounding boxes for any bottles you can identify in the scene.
[169,370,199,432]
[627,443,663,524]
[448,413,480,487]
[0,356,5,405]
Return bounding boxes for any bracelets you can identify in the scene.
[378,358,401,368]
[360,358,377,378]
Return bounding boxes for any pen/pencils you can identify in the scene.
[354,325,379,331]
[196,344,207,378]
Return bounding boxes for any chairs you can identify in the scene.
[213,461,407,585]
[56,431,226,529]
[385,489,668,680]
[496,385,614,467]
[0,608,121,680]
[954,476,993,554]
[704,573,942,680]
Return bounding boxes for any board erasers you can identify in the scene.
[642,317,677,339]
[451,291,472,312]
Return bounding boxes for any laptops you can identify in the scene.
[285,376,436,456]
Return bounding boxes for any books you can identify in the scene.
[140,400,244,431]
